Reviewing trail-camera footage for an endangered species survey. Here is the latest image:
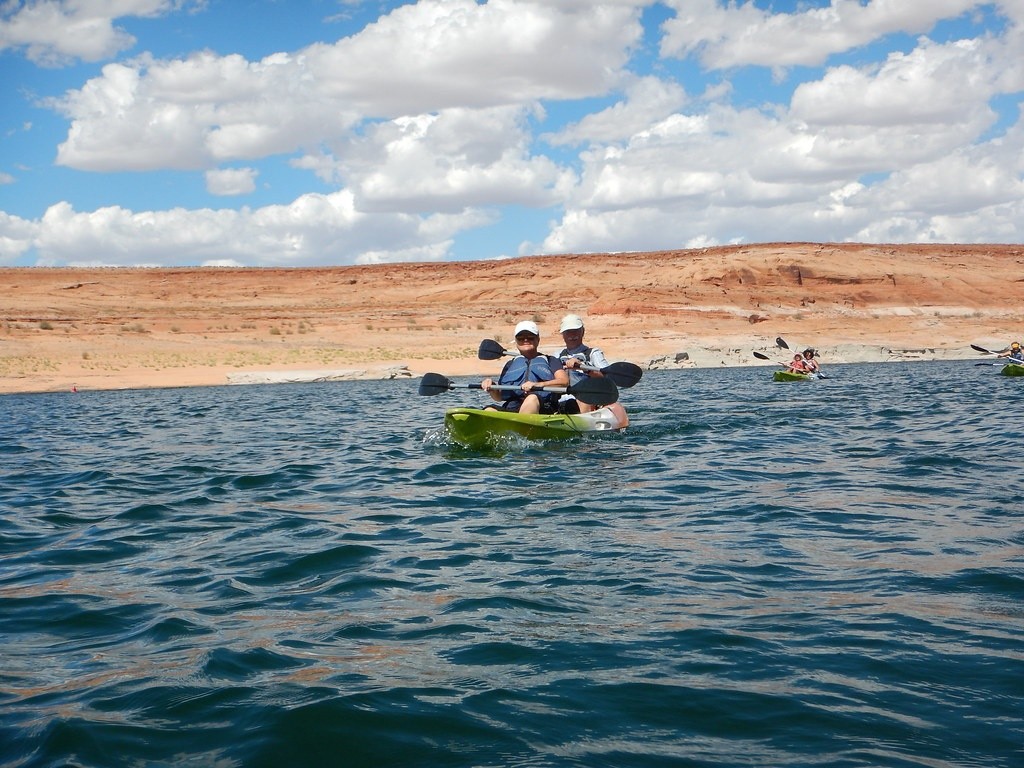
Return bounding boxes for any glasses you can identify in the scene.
[516,334,536,341]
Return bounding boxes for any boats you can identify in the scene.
[774,371,822,382]
[1000,364,1024,376]
[444,403,631,450]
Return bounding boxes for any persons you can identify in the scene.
[782,350,822,374]
[554,314,610,413]
[997,342,1024,366]
[482,321,570,415]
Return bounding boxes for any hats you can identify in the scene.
[804,350,815,358]
[515,321,538,336]
[1011,343,1019,348]
[559,314,583,333]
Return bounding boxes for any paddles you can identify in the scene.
[418,372,619,405]
[974,363,1024,366]
[775,337,829,379]
[970,344,1024,363]
[753,351,824,379]
[477,338,642,388]
